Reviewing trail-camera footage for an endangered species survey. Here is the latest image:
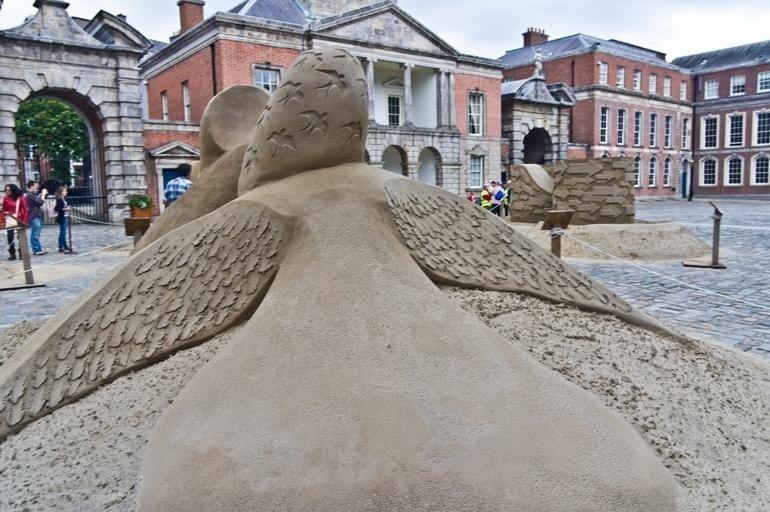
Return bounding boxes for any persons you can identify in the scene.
[466,180,512,217]
[162,163,193,203]
[0,180,74,260]
[530,46,552,76]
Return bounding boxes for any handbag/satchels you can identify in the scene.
[5,214,18,228]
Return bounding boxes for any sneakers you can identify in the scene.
[59,248,73,253]
[33,251,48,255]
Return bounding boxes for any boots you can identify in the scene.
[8,248,21,260]
